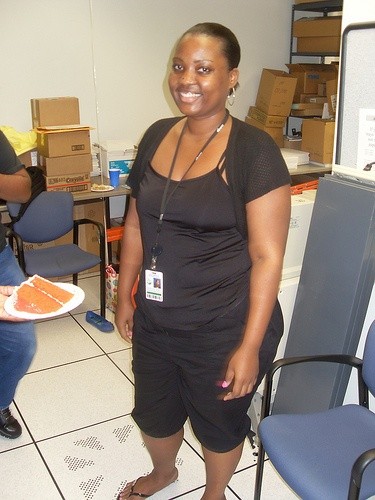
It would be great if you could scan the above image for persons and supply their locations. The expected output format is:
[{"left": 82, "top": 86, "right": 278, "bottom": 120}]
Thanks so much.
[
  {"left": 0, "top": 129, "right": 38, "bottom": 439},
  {"left": 154, "top": 280, "right": 161, "bottom": 288},
  {"left": 116, "top": 24, "right": 293, "bottom": 500}
]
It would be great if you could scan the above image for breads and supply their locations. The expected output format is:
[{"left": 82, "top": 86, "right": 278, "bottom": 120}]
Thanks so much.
[{"left": 92, "top": 184, "right": 109, "bottom": 190}]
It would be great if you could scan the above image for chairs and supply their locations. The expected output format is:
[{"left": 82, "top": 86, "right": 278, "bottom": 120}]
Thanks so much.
[
  {"left": 6, "top": 190, "right": 106, "bottom": 318},
  {"left": 253, "top": 321, "right": 375, "bottom": 500}
]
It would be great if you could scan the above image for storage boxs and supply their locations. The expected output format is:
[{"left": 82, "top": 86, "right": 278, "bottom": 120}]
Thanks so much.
[
  {"left": 30, "top": 96, "right": 92, "bottom": 195},
  {"left": 244, "top": 16, "right": 341, "bottom": 163}
]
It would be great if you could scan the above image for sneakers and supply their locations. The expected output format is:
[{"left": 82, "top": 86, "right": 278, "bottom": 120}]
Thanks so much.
[{"left": 0, "top": 409, "right": 21, "bottom": 439}]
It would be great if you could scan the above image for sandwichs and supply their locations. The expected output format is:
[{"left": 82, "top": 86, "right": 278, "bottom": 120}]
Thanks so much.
[{"left": 12, "top": 274, "right": 74, "bottom": 313}]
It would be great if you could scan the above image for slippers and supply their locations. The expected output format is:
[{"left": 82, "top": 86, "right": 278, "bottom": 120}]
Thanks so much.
[{"left": 116, "top": 467, "right": 178, "bottom": 500}]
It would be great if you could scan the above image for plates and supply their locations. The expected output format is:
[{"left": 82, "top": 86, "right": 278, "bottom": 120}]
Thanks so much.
[
  {"left": 3, "top": 282, "right": 85, "bottom": 319},
  {"left": 91, "top": 185, "right": 114, "bottom": 192}
]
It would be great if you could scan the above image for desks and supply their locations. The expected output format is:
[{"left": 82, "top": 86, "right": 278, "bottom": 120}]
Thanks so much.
[{"left": 0, "top": 189, "right": 130, "bottom": 266}]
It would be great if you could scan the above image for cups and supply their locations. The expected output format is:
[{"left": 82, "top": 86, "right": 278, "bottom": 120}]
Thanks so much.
[{"left": 109, "top": 169, "right": 120, "bottom": 189}]
